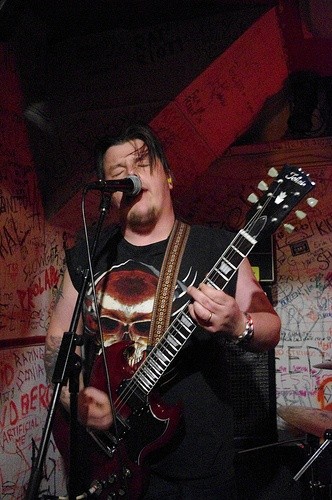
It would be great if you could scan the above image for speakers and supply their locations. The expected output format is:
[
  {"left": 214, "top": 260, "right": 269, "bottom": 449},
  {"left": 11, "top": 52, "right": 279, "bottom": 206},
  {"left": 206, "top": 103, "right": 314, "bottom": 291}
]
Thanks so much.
[{"left": 212, "top": 227, "right": 279, "bottom": 454}]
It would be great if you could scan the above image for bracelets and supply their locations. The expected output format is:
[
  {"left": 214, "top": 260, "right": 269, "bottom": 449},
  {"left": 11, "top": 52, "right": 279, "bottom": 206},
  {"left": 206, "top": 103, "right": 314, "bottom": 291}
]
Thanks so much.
[{"left": 226, "top": 311, "right": 254, "bottom": 347}]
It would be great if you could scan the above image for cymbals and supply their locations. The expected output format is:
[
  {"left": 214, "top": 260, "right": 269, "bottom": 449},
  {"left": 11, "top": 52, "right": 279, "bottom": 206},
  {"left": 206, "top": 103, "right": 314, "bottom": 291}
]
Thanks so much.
[
  {"left": 276, "top": 406, "right": 332, "bottom": 439},
  {"left": 314, "top": 360, "right": 332, "bottom": 371}
]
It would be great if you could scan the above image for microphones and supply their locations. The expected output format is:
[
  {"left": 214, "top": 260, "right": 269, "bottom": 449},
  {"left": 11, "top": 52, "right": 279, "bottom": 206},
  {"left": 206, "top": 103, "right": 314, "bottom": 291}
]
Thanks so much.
[{"left": 86, "top": 176, "right": 142, "bottom": 199}]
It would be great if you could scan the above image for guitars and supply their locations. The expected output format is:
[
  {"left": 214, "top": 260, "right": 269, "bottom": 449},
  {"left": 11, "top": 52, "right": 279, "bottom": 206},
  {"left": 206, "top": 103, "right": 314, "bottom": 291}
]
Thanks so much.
[{"left": 80, "top": 162, "right": 321, "bottom": 481}]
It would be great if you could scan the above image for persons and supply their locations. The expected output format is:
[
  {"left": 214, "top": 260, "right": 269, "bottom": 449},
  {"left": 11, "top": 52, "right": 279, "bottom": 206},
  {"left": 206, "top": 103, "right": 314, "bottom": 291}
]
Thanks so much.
[{"left": 43, "top": 122, "right": 281, "bottom": 500}]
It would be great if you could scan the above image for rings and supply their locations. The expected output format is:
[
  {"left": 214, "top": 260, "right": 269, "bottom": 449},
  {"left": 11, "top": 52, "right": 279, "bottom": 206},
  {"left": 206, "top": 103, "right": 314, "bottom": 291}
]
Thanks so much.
[{"left": 204, "top": 313, "right": 213, "bottom": 323}]
